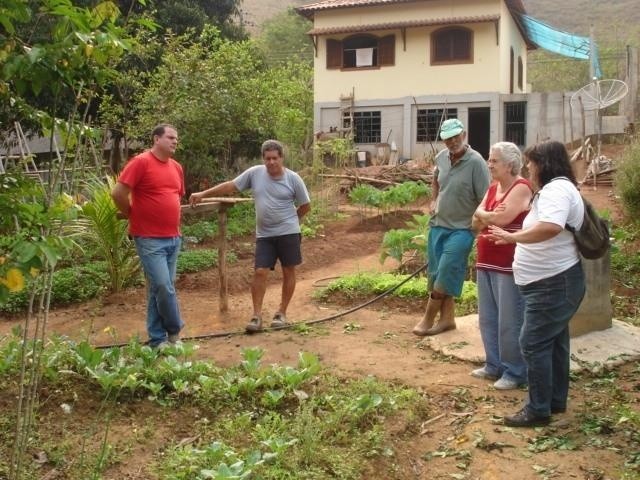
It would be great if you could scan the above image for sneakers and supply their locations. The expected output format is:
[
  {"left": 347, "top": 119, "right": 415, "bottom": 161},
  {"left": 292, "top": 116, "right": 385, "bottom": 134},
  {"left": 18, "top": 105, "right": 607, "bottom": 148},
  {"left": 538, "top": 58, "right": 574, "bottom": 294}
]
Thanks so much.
[
  {"left": 271, "top": 312, "right": 285, "bottom": 326},
  {"left": 158, "top": 340, "right": 182, "bottom": 351},
  {"left": 494, "top": 378, "right": 518, "bottom": 389},
  {"left": 247, "top": 316, "right": 261, "bottom": 330},
  {"left": 472, "top": 364, "right": 495, "bottom": 379}
]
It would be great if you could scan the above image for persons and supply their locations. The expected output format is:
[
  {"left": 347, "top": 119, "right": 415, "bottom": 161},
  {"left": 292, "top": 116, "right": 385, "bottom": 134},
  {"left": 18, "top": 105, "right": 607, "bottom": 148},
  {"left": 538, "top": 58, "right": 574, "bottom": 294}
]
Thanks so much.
[
  {"left": 111, "top": 123, "right": 186, "bottom": 356},
  {"left": 471, "top": 141, "right": 534, "bottom": 391},
  {"left": 412, "top": 118, "right": 491, "bottom": 337},
  {"left": 483, "top": 139, "right": 586, "bottom": 427},
  {"left": 189, "top": 139, "right": 311, "bottom": 332}
]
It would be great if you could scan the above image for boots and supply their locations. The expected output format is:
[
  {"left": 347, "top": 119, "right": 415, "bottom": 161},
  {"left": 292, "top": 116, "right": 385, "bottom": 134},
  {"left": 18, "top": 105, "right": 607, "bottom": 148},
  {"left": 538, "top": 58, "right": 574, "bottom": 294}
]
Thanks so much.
[
  {"left": 427, "top": 297, "right": 456, "bottom": 335},
  {"left": 413, "top": 297, "right": 441, "bottom": 336}
]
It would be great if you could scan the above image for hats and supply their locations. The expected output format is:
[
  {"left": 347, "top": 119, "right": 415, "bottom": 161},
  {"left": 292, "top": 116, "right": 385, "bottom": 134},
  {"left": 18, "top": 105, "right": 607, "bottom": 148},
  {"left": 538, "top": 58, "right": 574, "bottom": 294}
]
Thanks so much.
[{"left": 440, "top": 120, "right": 463, "bottom": 140}]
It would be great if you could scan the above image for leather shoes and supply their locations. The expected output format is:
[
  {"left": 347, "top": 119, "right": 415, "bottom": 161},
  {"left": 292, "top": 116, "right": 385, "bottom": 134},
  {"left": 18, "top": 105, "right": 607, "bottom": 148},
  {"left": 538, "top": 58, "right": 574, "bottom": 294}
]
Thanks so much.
[{"left": 505, "top": 409, "right": 549, "bottom": 426}]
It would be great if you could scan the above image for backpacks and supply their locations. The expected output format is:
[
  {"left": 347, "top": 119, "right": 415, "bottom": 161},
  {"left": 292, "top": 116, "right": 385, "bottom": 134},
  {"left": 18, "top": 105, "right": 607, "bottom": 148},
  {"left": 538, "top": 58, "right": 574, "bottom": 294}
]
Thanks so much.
[{"left": 538, "top": 178, "right": 608, "bottom": 259}]
